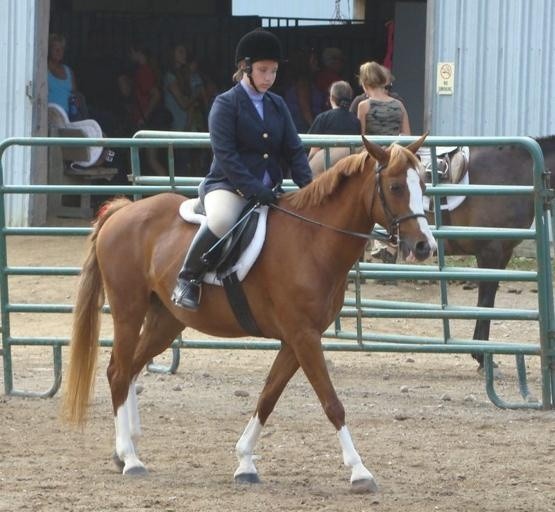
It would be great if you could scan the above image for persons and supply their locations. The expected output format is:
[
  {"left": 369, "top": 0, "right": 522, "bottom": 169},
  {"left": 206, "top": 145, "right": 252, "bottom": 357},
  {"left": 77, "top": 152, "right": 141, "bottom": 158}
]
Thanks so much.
[
  {"left": 317, "top": 48, "right": 353, "bottom": 95},
  {"left": 302, "top": 81, "right": 360, "bottom": 180},
  {"left": 357, "top": 62, "right": 412, "bottom": 151},
  {"left": 157, "top": 37, "right": 198, "bottom": 177},
  {"left": 171, "top": 30, "right": 312, "bottom": 311},
  {"left": 47, "top": 22, "right": 165, "bottom": 203},
  {"left": 282, "top": 47, "right": 321, "bottom": 133}
]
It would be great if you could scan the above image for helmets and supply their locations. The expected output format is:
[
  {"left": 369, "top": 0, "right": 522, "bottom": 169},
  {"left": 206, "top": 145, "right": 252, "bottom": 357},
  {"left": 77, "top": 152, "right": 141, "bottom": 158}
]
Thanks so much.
[{"left": 236, "top": 30, "right": 284, "bottom": 63}]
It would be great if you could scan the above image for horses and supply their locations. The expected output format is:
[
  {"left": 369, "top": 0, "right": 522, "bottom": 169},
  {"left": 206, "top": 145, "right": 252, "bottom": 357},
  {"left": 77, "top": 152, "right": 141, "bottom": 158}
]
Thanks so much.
[
  {"left": 309, "top": 133, "right": 555, "bottom": 378},
  {"left": 63, "top": 129, "right": 440, "bottom": 492}
]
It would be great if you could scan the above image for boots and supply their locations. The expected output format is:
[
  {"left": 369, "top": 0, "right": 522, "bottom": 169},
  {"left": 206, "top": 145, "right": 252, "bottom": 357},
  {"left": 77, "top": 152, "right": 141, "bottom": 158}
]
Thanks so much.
[{"left": 171, "top": 221, "right": 227, "bottom": 309}]
